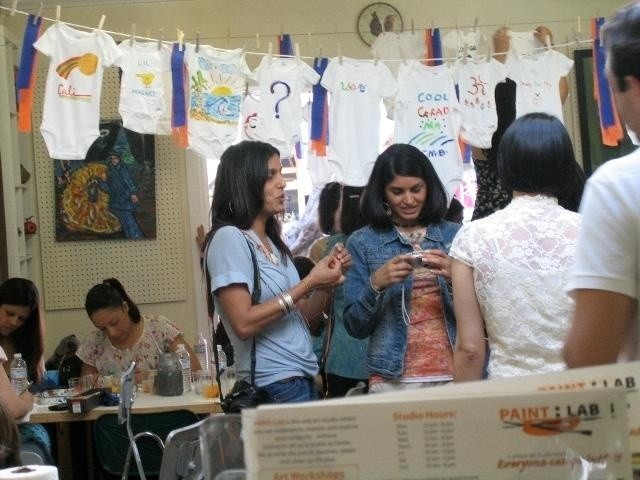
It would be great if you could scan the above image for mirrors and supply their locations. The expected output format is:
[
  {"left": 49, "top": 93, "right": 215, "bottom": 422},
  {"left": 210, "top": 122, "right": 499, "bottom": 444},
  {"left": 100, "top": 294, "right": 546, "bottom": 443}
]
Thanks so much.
[{"left": 574, "top": 46, "right": 640, "bottom": 182}]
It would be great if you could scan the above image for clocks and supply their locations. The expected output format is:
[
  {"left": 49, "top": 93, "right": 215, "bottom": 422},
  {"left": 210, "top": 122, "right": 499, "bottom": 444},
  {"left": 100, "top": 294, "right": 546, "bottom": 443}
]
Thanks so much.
[{"left": 356, "top": 2, "right": 405, "bottom": 48}]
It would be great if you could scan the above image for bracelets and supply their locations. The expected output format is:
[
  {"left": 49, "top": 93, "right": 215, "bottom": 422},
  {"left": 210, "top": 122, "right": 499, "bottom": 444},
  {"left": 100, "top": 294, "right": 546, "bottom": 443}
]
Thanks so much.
[
  {"left": 274, "top": 295, "right": 288, "bottom": 315},
  {"left": 280, "top": 291, "right": 294, "bottom": 312},
  {"left": 301, "top": 279, "right": 309, "bottom": 296}
]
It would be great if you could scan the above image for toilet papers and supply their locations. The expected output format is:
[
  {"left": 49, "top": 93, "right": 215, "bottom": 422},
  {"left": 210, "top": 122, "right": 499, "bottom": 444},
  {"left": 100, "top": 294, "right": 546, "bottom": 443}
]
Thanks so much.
[{"left": 0, "top": 464, "right": 59, "bottom": 480}]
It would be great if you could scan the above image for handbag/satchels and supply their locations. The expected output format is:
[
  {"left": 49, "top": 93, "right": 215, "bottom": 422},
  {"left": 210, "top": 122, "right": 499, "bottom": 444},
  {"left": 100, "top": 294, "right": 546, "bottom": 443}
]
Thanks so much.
[{"left": 205, "top": 225, "right": 267, "bottom": 414}]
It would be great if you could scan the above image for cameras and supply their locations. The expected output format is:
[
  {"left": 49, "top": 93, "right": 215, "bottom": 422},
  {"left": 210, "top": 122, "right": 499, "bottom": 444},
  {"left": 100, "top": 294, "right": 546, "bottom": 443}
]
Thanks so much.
[{"left": 405, "top": 251, "right": 430, "bottom": 270}]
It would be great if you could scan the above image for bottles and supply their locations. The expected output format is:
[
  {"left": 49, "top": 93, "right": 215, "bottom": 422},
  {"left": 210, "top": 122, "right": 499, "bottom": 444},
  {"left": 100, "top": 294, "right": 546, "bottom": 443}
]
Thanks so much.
[
  {"left": 176, "top": 344, "right": 192, "bottom": 393},
  {"left": 193, "top": 333, "right": 209, "bottom": 372},
  {"left": 158, "top": 352, "right": 183, "bottom": 396},
  {"left": 10, "top": 353, "right": 27, "bottom": 397},
  {"left": 210, "top": 344, "right": 228, "bottom": 388}
]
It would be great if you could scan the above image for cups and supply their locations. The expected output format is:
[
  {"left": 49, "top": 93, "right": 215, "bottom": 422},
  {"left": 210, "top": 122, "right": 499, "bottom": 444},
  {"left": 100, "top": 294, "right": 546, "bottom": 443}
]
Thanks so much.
[
  {"left": 83, "top": 375, "right": 123, "bottom": 393},
  {"left": 192, "top": 372, "right": 211, "bottom": 395},
  {"left": 143, "top": 370, "right": 159, "bottom": 393},
  {"left": 68, "top": 378, "right": 81, "bottom": 396},
  {"left": 200, "top": 375, "right": 219, "bottom": 398}
]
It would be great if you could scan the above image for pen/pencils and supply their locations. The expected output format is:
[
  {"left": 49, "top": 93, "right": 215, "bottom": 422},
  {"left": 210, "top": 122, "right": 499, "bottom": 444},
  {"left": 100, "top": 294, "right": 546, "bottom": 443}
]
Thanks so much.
[
  {"left": 19, "top": 381, "right": 34, "bottom": 395},
  {"left": 91, "top": 367, "right": 106, "bottom": 389}
]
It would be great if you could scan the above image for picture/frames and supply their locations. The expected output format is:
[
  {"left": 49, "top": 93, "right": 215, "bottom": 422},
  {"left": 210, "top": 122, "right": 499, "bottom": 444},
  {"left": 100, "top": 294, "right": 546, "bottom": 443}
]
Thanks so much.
[{"left": 52, "top": 120, "right": 158, "bottom": 243}]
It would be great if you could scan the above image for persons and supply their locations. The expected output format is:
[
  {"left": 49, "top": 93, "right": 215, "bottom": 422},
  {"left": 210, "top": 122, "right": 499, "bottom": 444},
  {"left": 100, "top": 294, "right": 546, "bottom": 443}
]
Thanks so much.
[
  {"left": 452, "top": 112, "right": 584, "bottom": 384},
  {"left": 207, "top": 141, "right": 352, "bottom": 404},
  {"left": 195, "top": 225, "right": 234, "bottom": 369},
  {"left": 561, "top": 2, "right": 640, "bottom": 369},
  {"left": 293, "top": 257, "right": 327, "bottom": 399},
  {"left": 0, "top": 343, "right": 50, "bottom": 470},
  {"left": 0, "top": 278, "right": 52, "bottom": 466},
  {"left": 73, "top": 277, "right": 203, "bottom": 480},
  {"left": 342, "top": 143, "right": 465, "bottom": 394},
  {"left": 323, "top": 186, "right": 373, "bottom": 397},
  {"left": 309, "top": 180, "right": 344, "bottom": 263},
  {"left": 85, "top": 152, "right": 143, "bottom": 239}
]
